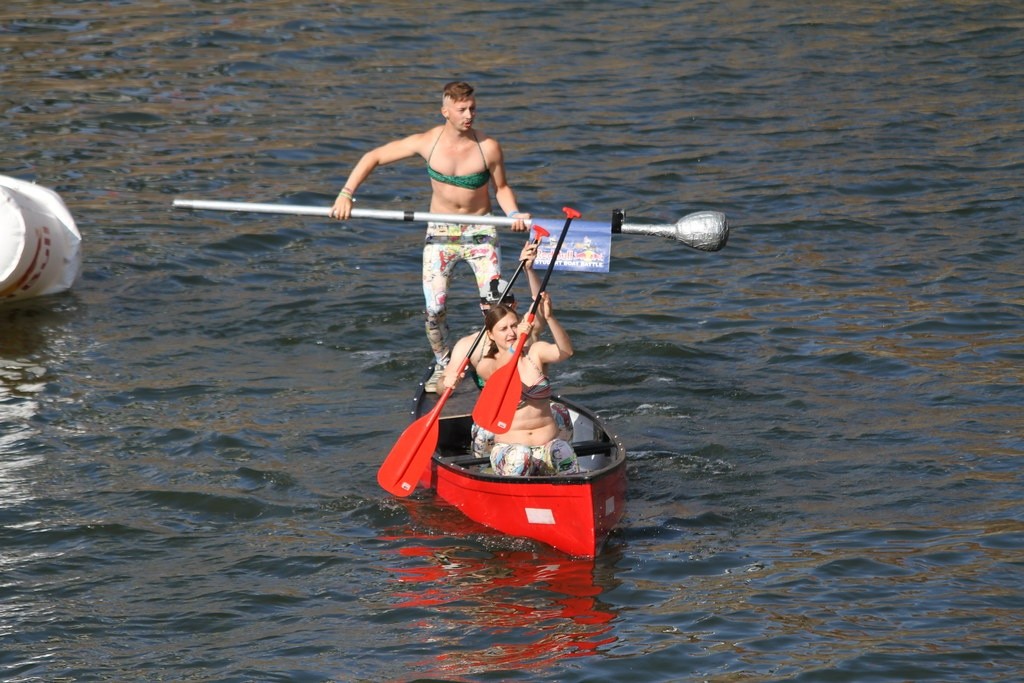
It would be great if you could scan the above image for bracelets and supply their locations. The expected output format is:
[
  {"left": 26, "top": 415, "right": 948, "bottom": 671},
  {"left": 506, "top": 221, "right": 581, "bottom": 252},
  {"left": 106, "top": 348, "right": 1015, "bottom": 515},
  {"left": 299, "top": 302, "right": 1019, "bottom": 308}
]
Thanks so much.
[
  {"left": 338, "top": 186, "right": 356, "bottom": 203},
  {"left": 509, "top": 211, "right": 520, "bottom": 217},
  {"left": 509, "top": 345, "right": 515, "bottom": 354}
]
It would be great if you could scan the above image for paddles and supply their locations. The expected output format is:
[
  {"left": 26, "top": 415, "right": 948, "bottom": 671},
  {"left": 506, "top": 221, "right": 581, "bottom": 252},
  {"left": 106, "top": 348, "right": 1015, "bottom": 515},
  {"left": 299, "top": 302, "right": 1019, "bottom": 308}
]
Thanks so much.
[
  {"left": 375, "top": 225, "right": 550, "bottom": 498},
  {"left": 471, "top": 207, "right": 581, "bottom": 436}
]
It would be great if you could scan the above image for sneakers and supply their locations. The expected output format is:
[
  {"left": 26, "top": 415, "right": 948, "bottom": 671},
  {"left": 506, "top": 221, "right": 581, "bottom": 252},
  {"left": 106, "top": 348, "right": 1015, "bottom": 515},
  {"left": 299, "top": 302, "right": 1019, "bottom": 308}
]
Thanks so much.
[{"left": 424, "top": 361, "right": 450, "bottom": 392}]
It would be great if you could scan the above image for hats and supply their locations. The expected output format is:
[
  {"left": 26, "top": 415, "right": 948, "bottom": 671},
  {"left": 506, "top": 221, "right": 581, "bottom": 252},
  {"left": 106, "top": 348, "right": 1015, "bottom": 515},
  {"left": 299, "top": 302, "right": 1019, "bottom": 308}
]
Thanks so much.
[{"left": 480, "top": 278, "right": 515, "bottom": 304}]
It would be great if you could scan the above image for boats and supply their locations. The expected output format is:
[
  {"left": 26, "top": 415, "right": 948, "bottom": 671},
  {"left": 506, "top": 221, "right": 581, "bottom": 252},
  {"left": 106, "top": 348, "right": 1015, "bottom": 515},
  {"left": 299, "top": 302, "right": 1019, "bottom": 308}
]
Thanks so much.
[{"left": 412, "top": 355, "right": 622, "bottom": 559}]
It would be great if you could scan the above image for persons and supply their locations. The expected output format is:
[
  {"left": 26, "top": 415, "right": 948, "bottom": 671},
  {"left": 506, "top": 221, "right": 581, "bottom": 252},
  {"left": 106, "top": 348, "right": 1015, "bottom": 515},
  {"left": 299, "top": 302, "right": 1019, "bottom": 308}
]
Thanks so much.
[
  {"left": 437, "top": 238, "right": 578, "bottom": 475},
  {"left": 329, "top": 82, "right": 531, "bottom": 393}
]
